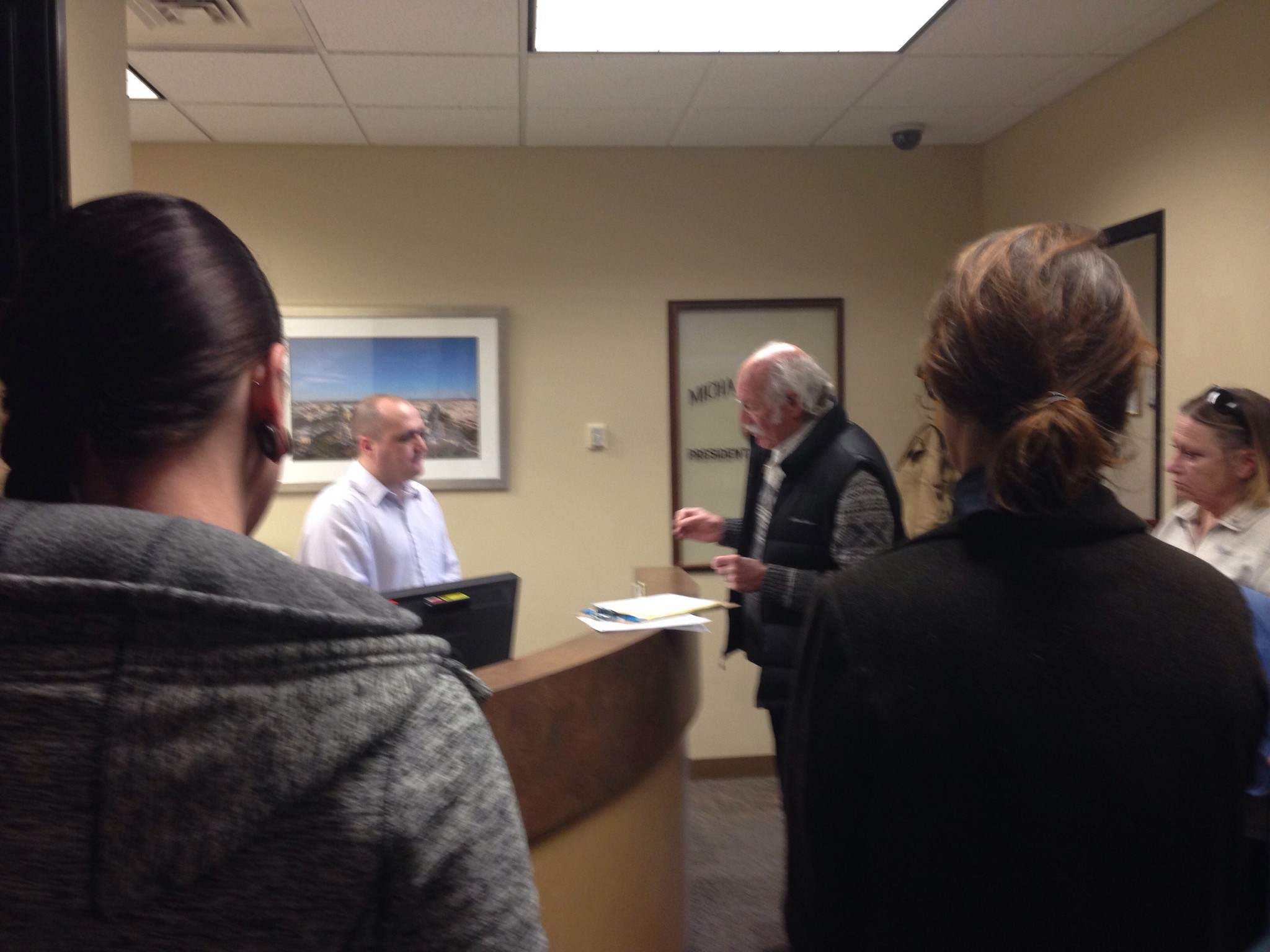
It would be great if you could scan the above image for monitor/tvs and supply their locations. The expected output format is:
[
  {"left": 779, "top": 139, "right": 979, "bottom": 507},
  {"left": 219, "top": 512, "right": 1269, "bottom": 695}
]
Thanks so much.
[{"left": 378, "top": 572, "right": 520, "bottom": 669}]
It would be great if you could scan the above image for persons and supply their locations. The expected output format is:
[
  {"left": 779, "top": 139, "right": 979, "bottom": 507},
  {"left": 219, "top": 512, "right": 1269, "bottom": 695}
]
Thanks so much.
[
  {"left": 0, "top": 192, "right": 546, "bottom": 952},
  {"left": 681, "top": 338, "right": 910, "bottom": 664},
  {"left": 758, "top": 223, "right": 1270, "bottom": 952},
  {"left": 298, "top": 394, "right": 463, "bottom": 609},
  {"left": 1149, "top": 386, "right": 1270, "bottom": 682}
]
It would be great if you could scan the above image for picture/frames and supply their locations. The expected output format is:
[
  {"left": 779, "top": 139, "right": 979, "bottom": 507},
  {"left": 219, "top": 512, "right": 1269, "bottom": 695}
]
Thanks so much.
[
  {"left": 273, "top": 306, "right": 508, "bottom": 493},
  {"left": 668, "top": 297, "right": 845, "bottom": 573},
  {"left": 1096, "top": 208, "right": 1165, "bottom": 523}
]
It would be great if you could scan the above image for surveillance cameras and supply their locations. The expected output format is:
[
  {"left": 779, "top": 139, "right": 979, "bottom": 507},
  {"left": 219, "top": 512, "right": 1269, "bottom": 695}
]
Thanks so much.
[{"left": 890, "top": 122, "right": 924, "bottom": 151}]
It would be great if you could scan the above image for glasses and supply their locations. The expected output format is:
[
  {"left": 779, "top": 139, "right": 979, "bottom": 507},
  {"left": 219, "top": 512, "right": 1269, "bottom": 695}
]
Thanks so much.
[
  {"left": 1207, "top": 385, "right": 1249, "bottom": 430},
  {"left": 735, "top": 398, "right": 790, "bottom": 417}
]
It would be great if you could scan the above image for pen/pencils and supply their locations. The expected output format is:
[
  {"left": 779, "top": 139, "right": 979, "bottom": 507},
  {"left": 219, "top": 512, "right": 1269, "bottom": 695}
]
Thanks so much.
[{"left": 580, "top": 608, "right": 644, "bottom": 623}]
